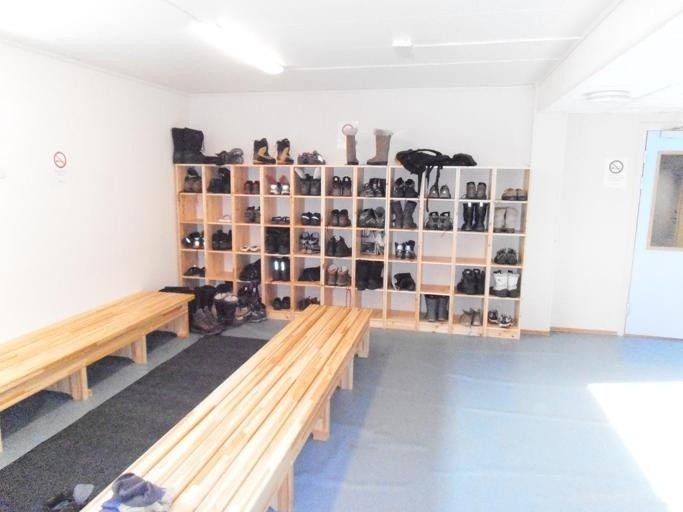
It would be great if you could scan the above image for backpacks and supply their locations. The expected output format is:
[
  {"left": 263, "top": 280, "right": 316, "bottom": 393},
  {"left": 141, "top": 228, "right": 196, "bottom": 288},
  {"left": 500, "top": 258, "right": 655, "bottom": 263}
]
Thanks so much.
[{"left": 395, "top": 148, "right": 476, "bottom": 212}]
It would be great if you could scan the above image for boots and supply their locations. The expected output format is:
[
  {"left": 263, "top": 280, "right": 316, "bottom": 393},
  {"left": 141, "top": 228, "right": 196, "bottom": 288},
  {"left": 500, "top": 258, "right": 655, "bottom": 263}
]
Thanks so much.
[
  {"left": 170, "top": 123, "right": 392, "bottom": 166},
  {"left": 181, "top": 168, "right": 525, "bottom": 335}
]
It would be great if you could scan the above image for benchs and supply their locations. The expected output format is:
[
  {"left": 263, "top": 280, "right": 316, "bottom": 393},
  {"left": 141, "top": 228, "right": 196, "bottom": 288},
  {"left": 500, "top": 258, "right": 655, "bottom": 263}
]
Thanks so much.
[
  {"left": 0, "top": 290, "right": 195, "bottom": 453},
  {"left": 80, "top": 304, "right": 373, "bottom": 512}
]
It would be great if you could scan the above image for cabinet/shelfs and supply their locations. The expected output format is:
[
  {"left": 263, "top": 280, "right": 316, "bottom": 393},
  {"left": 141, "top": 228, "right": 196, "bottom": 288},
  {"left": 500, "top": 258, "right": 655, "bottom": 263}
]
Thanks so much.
[{"left": 173, "top": 163, "right": 532, "bottom": 340}]
